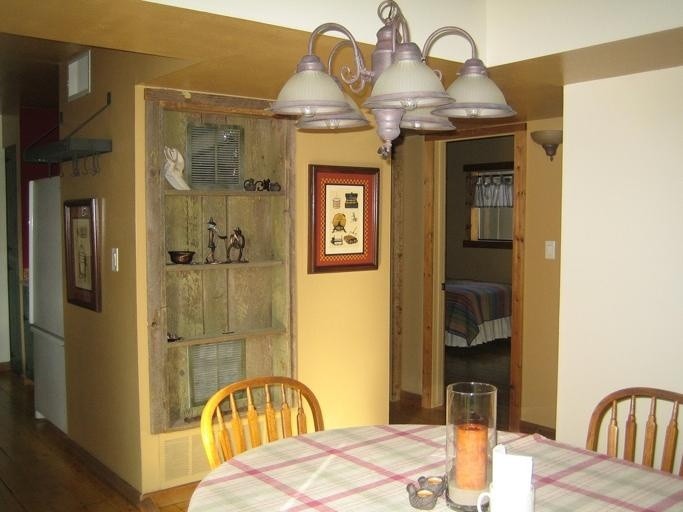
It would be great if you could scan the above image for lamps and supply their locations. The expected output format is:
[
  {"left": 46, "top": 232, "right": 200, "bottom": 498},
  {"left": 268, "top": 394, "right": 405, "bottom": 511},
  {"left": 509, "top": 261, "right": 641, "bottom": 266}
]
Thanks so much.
[
  {"left": 529, "top": 129, "right": 561, "bottom": 160},
  {"left": 271, "top": 0, "right": 516, "bottom": 159}
]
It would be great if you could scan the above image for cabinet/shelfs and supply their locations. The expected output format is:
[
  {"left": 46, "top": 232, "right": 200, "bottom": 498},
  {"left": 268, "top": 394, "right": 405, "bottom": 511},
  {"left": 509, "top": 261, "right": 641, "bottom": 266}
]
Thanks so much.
[{"left": 142, "top": 86, "right": 297, "bottom": 435}]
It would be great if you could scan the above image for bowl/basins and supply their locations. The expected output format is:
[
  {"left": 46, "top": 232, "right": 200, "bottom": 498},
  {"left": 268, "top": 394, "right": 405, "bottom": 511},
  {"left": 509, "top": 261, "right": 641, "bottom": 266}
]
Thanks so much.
[{"left": 168, "top": 251, "right": 197, "bottom": 265}]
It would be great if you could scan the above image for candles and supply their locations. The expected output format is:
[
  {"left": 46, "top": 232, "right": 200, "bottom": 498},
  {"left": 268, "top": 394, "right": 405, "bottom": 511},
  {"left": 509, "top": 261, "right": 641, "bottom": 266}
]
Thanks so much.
[{"left": 455, "top": 423, "right": 487, "bottom": 491}]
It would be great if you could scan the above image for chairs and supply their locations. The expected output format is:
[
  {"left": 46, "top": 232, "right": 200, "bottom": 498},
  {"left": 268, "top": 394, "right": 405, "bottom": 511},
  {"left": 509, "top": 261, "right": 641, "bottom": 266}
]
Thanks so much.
[
  {"left": 584, "top": 386, "right": 682, "bottom": 478},
  {"left": 199, "top": 375, "right": 323, "bottom": 468}
]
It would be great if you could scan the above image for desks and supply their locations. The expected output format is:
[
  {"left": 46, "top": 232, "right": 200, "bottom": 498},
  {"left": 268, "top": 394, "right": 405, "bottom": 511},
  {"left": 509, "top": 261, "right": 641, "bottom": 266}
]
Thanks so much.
[{"left": 187, "top": 423, "right": 683, "bottom": 512}]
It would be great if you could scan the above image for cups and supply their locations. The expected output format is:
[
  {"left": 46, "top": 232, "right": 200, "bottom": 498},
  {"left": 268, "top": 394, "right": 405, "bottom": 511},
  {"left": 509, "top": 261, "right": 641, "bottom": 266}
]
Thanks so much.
[
  {"left": 477, "top": 484, "right": 535, "bottom": 511},
  {"left": 447, "top": 383, "right": 497, "bottom": 512}
]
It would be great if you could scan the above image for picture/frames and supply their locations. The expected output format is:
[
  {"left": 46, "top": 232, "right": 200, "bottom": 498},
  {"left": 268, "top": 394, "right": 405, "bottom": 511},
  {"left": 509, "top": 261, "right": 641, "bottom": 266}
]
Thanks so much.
[
  {"left": 61, "top": 196, "right": 102, "bottom": 314},
  {"left": 306, "top": 163, "right": 380, "bottom": 275}
]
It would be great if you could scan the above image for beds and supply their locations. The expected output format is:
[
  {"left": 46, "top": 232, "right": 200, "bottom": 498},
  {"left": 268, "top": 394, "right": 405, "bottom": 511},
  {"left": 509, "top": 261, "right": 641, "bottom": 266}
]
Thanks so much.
[{"left": 444, "top": 280, "right": 512, "bottom": 365}]
